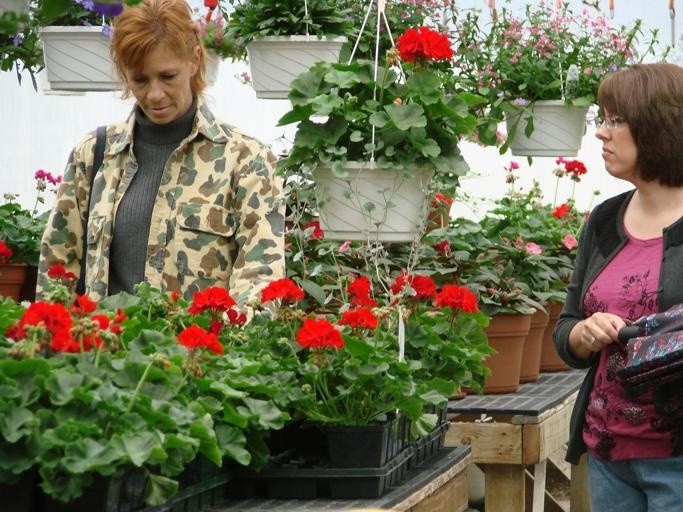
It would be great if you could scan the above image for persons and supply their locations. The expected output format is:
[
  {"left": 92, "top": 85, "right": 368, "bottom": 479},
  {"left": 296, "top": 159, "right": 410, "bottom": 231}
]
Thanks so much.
[
  {"left": 33, "top": 2, "right": 289, "bottom": 321},
  {"left": 550, "top": 60, "right": 682, "bottom": 511}
]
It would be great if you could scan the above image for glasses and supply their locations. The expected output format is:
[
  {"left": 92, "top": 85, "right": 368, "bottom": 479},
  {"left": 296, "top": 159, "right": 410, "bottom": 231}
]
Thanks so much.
[{"left": 594, "top": 114, "right": 627, "bottom": 130}]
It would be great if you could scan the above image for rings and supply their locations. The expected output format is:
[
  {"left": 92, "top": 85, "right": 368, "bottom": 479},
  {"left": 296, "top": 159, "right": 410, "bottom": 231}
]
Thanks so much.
[{"left": 589, "top": 336, "right": 597, "bottom": 346}]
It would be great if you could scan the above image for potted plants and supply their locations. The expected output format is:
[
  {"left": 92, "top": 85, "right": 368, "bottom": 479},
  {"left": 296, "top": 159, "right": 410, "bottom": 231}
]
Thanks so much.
[
  {"left": 3, "top": 275, "right": 500, "bottom": 482},
  {"left": 0, "top": 158, "right": 604, "bottom": 394},
  {"left": 225, "top": 1, "right": 349, "bottom": 101}
]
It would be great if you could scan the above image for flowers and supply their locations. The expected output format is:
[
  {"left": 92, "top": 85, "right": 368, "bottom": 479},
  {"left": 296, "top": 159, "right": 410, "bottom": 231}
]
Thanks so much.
[
  {"left": 461, "top": 1, "right": 666, "bottom": 155},
  {"left": 273, "top": 26, "right": 497, "bottom": 182},
  {"left": 2, "top": 0, "right": 146, "bottom": 91}
]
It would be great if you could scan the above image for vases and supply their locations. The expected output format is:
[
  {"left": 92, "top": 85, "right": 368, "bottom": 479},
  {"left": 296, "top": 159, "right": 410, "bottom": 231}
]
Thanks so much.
[
  {"left": 301, "top": 159, "right": 440, "bottom": 244},
  {"left": 7, "top": 404, "right": 452, "bottom": 508},
  {"left": 494, "top": 93, "right": 593, "bottom": 158},
  {"left": 37, "top": 23, "right": 136, "bottom": 92}
]
